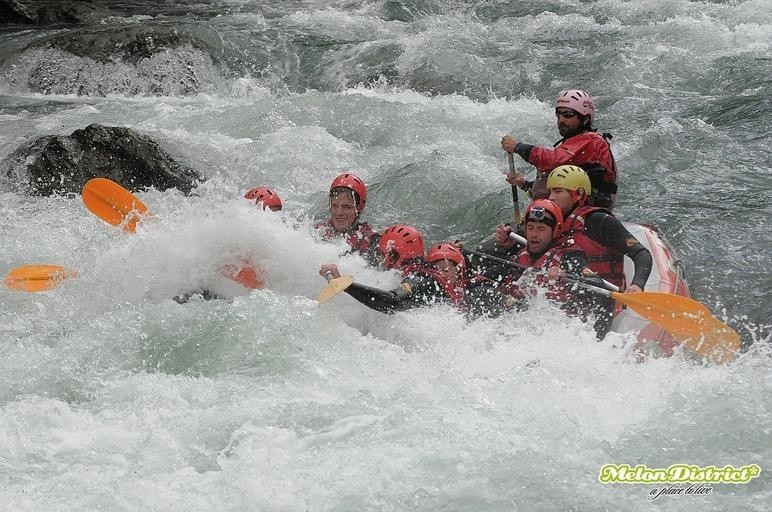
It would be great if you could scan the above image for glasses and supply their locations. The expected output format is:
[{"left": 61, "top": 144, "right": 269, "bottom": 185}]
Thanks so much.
[{"left": 555, "top": 109, "right": 577, "bottom": 118}]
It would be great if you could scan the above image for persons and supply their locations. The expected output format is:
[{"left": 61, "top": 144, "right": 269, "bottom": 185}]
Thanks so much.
[
  {"left": 497, "top": 198, "right": 609, "bottom": 342},
  {"left": 495, "top": 165, "right": 653, "bottom": 310},
  {"left": 313, "top": 174, "right": 373, "bottom": 256},
  {"left": 501, "top": 89, "right": 618, "bottom": 212},
  {"left": 423, "top": 243, "right": 507, "bottom": 321},
  {"left": 319, "top": 225, "right": 465, "bottom": 315}
]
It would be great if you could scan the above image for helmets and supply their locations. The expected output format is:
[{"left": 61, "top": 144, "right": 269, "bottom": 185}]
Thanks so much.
[
  {"left": 525, "top": 198, "right": 563, "bottom": 239},
  {"left": 546, "top": 164, "right": 592, "bottom": 196},
  {"left": 330, "top": 173, "right": 367, "bottom": 213},
  {"left": 377, "top": 224, "right": 425, "bottom": 264},
  {"left": 554, "top": 89, "right": 595, "bottom": 125},
  {"left": 427, "top": 243, "right": 466, "bottom": 272}
]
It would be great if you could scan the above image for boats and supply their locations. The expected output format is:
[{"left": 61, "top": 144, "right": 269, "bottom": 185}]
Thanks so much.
[{"left": 602, "top": 217, "right": 690, "bottom": 363}]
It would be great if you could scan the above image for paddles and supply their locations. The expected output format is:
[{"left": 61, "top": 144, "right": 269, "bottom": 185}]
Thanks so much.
[
  {"left": 464, "top": 245, "right": 713, "bottom": 336},
  {"left": 319, "top": 266, "right": 354, "bottom": 304},
  {"left": 83, "top": 179, "right": 151, "bottom": 235},
  {"left": 509, "top": 231, "right": 742, "bottom": 361},
  {"left": 5, "top": 265, "right": 64, "bottom": 292}
]
